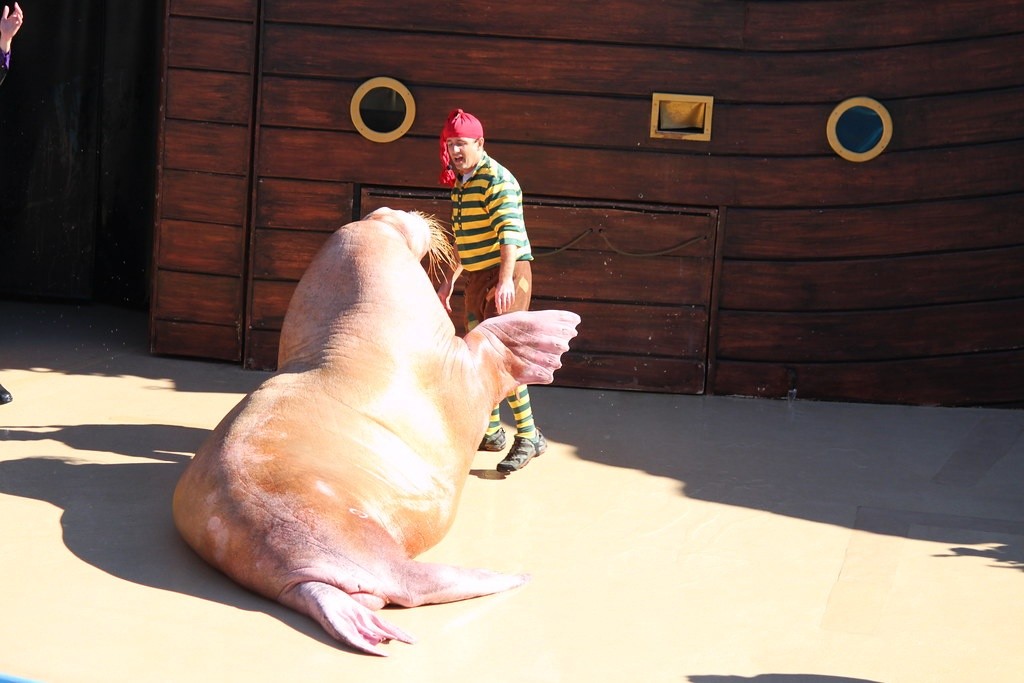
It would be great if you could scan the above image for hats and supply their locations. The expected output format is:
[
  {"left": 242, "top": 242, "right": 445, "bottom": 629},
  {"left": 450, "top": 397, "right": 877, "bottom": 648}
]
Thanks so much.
[{"left": 440, "top": 109, "right": 483, "bottom": 188}]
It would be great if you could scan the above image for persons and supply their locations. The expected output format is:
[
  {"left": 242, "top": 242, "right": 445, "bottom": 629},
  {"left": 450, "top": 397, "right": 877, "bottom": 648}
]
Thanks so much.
[
  {"left": 0, "top": 2, "right": 23, "bottom": 403},
  {"left": 438, "top": 108, "right": 546, "bottom": 472}
]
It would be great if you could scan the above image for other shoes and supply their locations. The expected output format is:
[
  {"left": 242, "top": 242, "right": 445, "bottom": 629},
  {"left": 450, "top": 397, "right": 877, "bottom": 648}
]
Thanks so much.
[
  {"left": 497, "top": 428, "right": 547, "bottom": 472},
  {"left": 477, "top": 427, "right": 506, "bottom": 451}
]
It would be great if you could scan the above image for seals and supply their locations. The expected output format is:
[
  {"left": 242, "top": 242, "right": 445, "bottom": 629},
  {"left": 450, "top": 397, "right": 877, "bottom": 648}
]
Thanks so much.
[{"left": 168, "top": 205, "right": 585, "bottom": 657}]
